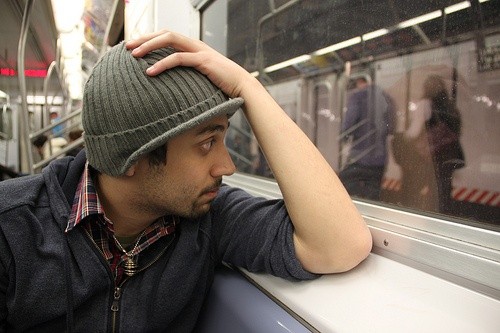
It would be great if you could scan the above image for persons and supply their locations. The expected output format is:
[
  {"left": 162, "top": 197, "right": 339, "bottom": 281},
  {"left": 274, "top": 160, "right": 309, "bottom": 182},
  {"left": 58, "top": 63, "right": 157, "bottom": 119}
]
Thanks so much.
[
  {"left": 0, "top": 28, "right": 373, "bottom": 333},
  {"left": 32, "top": 129, "right": 84, "bottom": 173},
  {"left": 337, "top": 69, "right": 398, "bottom": 202},
  {"left": 403, "top": 74, "right": 466, "bottom": 215}
]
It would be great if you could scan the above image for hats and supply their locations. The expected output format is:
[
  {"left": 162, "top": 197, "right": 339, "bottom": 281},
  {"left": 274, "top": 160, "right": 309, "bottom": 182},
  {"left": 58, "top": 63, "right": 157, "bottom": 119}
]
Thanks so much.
[
  {"left": 346, "top": 66, "right": 374, "bottom": 90},
  {"left": 418, "top": 75, "right": 445, "bottom": 99},
  {"left": 83, "top": 40, "right": 244, "bottom": 176}
]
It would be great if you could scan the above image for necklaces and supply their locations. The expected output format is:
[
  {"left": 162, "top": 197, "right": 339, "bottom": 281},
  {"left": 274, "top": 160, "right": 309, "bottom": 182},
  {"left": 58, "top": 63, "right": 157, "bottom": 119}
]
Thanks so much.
[{"left": 97, "top": 218, "right": 150, "bottom": 277}]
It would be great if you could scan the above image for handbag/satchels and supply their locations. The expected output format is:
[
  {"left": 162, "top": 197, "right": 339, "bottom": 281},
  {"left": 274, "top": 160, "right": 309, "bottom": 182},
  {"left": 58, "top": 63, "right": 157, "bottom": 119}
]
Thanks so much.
[{"left": 431, "top": 141, "right": 465, "bottom": 174}]
